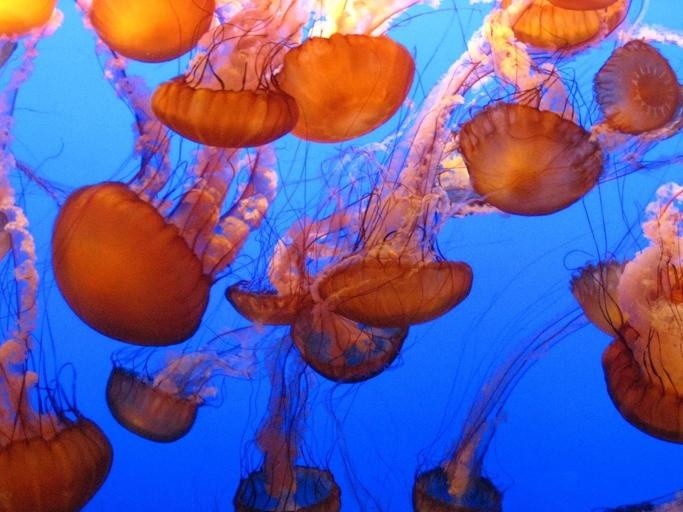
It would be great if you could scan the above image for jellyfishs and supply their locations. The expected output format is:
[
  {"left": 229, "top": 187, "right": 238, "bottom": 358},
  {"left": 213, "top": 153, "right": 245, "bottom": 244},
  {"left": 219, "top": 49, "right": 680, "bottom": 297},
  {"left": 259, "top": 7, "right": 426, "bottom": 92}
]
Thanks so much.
[{"left": 0, "top": 0, "right": 683, "bottom": 512}]
